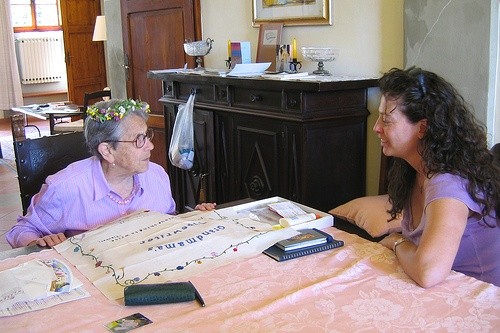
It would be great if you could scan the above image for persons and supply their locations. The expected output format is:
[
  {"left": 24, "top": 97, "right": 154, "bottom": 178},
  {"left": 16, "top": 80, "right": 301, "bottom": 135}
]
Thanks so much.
[
  {"left": 6, "top": 99, "right": 216, "bottom": 247},
  {"left": 373, "top": 66, "right": 500, "bottom": 289}
]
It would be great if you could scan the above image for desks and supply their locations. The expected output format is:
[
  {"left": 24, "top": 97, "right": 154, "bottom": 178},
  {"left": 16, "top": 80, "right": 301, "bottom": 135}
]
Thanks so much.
[
  {"left": 0, "top": 197, "right": 500, "bottom": 333},
  {"left": 9, "top": 102, "right": 83, "bottom": 141}
]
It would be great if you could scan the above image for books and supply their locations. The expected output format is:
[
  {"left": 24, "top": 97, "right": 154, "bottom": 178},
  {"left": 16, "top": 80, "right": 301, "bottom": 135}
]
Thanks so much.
[
  {"left": 201, "top": 62, "right": 308, "bottom": 79},
  {"left": 262, "top": 229, "right": 344, "bottom": 262},
  {"left": 256, "top": 24, "right": 283, "bottom": 72},
  {"left": 230, "top": 42, "right": 251, "bottom": 69}
]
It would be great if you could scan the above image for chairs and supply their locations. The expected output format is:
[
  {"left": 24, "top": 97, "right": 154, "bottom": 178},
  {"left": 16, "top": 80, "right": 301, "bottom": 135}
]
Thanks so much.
[
  {"left": 47, "top": 91, "right": 112, "bottom": 135},
  {"left": 13, "top": 132, "right": 92, "bottom": 216}
]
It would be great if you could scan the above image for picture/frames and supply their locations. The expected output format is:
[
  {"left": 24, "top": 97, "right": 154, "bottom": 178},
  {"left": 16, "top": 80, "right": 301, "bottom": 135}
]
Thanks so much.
[{"left": 252, "top": 0, "right": 334, "bottom": 28}]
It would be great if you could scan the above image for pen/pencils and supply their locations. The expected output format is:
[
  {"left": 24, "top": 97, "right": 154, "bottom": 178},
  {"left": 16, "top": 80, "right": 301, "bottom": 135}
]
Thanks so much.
[{"left": 188, "top": 280, "right": 205, "bottom": 307}]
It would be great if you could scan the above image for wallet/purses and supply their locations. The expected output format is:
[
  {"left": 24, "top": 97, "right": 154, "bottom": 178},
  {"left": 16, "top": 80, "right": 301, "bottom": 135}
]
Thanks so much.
[{"left": 124, "top": 282, "right": 194, "bottom": 306}]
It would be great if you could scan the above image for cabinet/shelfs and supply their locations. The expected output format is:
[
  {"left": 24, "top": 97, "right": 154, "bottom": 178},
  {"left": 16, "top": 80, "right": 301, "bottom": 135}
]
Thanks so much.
[{"left": 147, "top": 69, "right": 381, "bottom": 217}]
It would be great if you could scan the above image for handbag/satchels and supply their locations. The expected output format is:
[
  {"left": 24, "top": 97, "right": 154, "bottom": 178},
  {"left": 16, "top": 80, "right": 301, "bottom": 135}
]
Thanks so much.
[{"left": 168, "top": 92, "right": 195, "bottom": 170}]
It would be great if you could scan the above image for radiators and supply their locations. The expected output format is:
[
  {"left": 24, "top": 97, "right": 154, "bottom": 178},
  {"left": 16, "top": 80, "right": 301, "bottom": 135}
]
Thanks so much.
[{"left": 15, "top": 34, "right": 64, "bottom": 84}]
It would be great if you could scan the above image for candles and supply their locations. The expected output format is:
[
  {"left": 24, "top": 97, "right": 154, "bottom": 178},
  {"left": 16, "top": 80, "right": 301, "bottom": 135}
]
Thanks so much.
[{"left": 293, "top": 38, "right": 297, "bottom": 58}]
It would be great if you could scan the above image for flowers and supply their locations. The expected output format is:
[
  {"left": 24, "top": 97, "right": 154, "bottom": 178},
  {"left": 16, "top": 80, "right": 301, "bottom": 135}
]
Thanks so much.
[{"left": 86, "top": 98, "right": 151, "bottom": 123}]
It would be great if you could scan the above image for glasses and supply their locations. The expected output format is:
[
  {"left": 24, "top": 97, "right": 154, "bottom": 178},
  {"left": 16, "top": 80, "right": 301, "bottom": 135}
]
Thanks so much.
[{"left": 100, "top": 128, "right": 154, "bottom": 148}]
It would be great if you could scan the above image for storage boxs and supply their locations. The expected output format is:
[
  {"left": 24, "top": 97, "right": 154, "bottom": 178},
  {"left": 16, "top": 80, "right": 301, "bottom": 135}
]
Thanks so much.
[{"left": 218, "top": 196, "right": 334, "bottom": 230}]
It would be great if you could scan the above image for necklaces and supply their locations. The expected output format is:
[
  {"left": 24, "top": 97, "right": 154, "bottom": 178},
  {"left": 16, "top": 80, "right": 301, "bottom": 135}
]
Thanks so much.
[{"left": 107, "top": 194, "right": 135, "bottom": 204}]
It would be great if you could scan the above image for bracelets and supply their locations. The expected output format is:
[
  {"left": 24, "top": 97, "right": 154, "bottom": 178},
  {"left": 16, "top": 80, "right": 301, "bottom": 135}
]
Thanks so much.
[{"left": 393, "top": 238, "right": 412, "bottom": 255}]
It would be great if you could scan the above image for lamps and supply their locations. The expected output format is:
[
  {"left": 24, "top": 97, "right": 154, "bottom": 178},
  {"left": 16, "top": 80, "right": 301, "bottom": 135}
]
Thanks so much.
[{"left": 92, "top": 15, "right": 107, "bottom": 41}]
